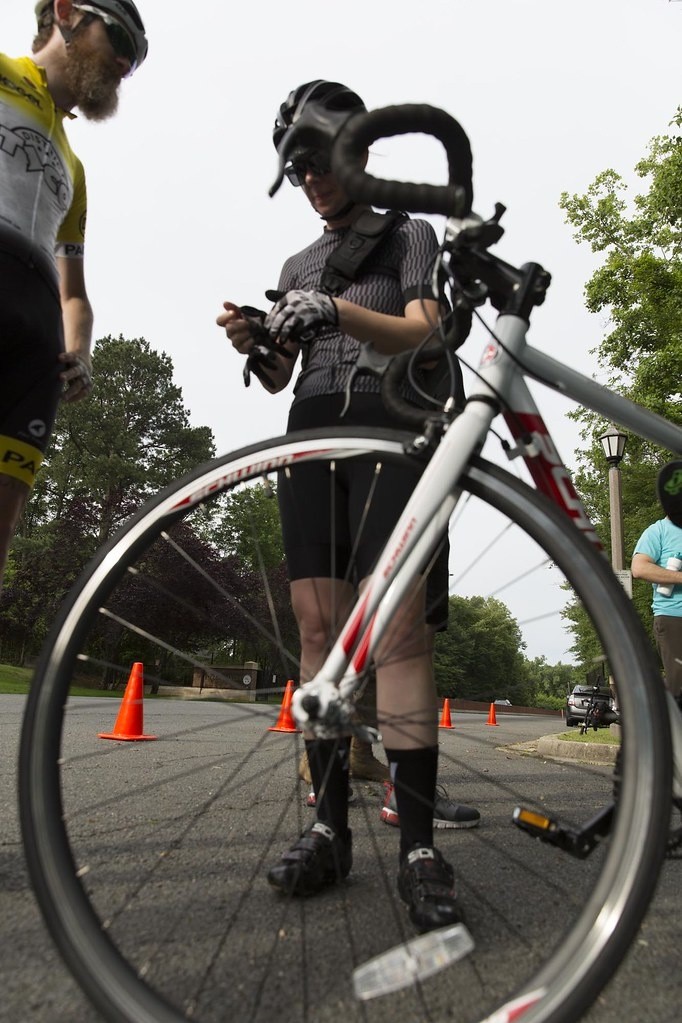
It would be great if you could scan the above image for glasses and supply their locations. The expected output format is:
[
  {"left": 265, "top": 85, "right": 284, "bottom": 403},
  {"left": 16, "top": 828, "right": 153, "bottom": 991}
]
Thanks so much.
[
  {"left": 285, "top": 143, "right": 337, "bottom": 187},
  {"left": 71, "top": 3, "right": 138, "bottom": 78}
]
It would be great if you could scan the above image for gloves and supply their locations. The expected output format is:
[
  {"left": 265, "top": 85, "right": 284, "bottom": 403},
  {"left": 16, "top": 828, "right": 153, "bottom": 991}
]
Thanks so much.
[
  {"left": 236, "top": 305, "right": 295, "bottom": 395},
  {"left": 263, "top": 289, "right": 340, "bottom": 345}
]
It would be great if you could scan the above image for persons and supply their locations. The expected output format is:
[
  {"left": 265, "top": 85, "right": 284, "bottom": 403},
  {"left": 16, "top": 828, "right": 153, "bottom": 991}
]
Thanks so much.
[
  {"left": 214, "top": 80, "right": 480, "bottom": 933},
  {"left": 630, "top": 515, "right": 682, "bottom": 713},
  {"left": 0, "top": 0, "right": 148, "bottom": 564}
]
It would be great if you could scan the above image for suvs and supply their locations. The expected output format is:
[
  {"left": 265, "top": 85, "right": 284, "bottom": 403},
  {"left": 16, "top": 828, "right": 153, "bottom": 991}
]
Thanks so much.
[{"left": 566, "top": 685, "right": 615, "bottom": 728}]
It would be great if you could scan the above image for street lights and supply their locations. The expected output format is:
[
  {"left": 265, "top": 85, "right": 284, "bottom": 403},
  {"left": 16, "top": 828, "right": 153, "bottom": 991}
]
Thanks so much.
[{"left": 596, "top": 421, "right": 627, "bottom": 574}]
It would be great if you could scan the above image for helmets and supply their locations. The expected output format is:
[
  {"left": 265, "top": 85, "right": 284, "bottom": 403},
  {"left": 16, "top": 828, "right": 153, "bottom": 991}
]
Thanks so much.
[
  {"left": 272, "top": 78, "right": 369, "bottom": 158},
  {"left": 35, "top": 0, "right": 148, "bottom": 68}
]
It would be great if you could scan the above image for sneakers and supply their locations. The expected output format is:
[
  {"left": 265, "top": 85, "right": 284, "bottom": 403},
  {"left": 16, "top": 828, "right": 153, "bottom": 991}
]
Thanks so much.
[
  {"left": 380, "top": 780, "right": 480, "bottom": 828},
  {"left": 266, "top": 823, "right": 353, "bottom": 890},
  {"left": 306, "top": 786, "right": 358, "bottom": 804},
  {"left": 396, "top": 843, "right": 466, "bottom": 932}
]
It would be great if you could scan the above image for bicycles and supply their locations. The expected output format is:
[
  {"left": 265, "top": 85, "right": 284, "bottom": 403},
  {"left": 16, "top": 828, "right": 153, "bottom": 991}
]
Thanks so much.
[{"left": 13, "top": 98, "right": 682, "bottom": 1023}]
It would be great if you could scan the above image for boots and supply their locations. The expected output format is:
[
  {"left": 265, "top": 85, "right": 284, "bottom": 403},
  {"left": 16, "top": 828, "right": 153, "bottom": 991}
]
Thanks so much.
[{"left": 352, "top": 742, "right": 394, "bottom": 783}]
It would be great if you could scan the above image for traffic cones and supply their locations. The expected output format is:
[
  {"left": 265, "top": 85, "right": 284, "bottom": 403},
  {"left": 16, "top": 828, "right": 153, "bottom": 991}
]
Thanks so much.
[
  {"left": 439, "top": 699, "right": 455, "bottom": 729},
  {"left": 485, "top": 703, "right": 502, "bottom": 730},
  {"left": 98, "top": 662, "right": 157, "bottom": 744},
  {"left": 267, "top": 679, "right": 303, "bottom": 733}
]
